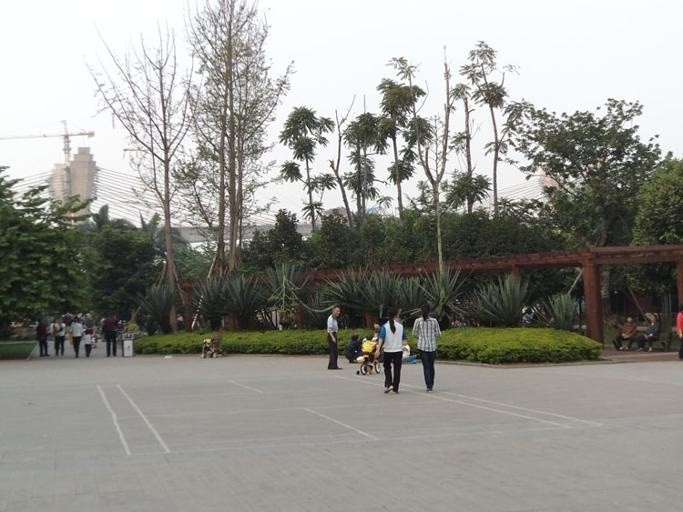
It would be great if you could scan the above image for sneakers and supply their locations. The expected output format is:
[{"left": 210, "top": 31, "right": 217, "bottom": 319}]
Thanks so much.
[{"left": 384, "top": 385, "right": 393, "bottom": 393}]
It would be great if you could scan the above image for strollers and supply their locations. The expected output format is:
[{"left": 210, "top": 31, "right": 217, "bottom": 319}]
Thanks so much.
[
  {"left": 356, "top": 337, "right": 383, "bottom": 375},
  {"left": 200, "top": 328, "right": 227, "bottom": 360}
]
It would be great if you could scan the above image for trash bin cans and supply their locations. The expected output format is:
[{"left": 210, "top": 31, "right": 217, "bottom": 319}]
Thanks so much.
[{"left": 121, "top": 332, "right": 134, "bottom": 357}]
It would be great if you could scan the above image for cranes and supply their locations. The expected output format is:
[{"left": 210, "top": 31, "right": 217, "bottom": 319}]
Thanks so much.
[{"left": 0, "top": 120, "right": 95, "bottom": 163}]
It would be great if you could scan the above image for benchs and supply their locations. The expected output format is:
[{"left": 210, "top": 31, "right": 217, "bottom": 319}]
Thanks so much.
[{"left": 632, "top": 325, "right": 673, "bottom": 352}]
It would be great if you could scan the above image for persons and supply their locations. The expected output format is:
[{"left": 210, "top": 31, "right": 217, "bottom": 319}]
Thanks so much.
[
  {"left": 326, "top": 303, "right": 441, "bottom": 394},
  {"left": 37, "top": 312, "right": 117, "bottom": 356},
  {"left": 615, "top": 303, "right": 683, "bottom": 359}
]
